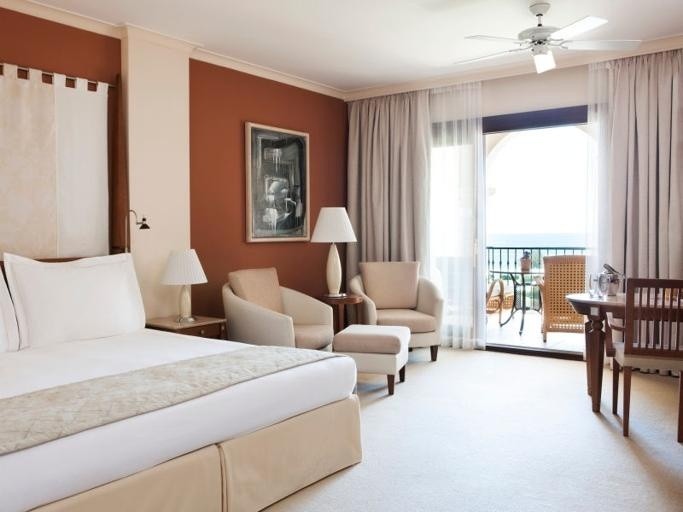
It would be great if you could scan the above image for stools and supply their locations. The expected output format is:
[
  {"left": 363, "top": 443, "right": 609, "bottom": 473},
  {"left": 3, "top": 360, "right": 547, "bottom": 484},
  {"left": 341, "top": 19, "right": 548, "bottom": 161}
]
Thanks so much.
[{"left": 329, "top": 322, "right": 409, "bottom": 395}]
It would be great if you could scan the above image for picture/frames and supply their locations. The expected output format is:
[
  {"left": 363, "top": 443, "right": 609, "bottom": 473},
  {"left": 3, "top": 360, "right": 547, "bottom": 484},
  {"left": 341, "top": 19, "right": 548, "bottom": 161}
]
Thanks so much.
[{"left": 242, "top": 120, "right": 311, "bottom": 245}]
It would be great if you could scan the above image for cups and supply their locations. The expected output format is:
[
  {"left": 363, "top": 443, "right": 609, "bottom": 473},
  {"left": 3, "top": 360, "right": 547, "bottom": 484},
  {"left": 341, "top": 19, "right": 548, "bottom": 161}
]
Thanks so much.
[
  {"left": 520, "top": 258, "right": 531, "bottom": 272},
  {"left": 587, "top": 271, "right": 597, "bottom": 298},
  {"left": 596, "top": 272, "right": 612, "bottom": 300}
]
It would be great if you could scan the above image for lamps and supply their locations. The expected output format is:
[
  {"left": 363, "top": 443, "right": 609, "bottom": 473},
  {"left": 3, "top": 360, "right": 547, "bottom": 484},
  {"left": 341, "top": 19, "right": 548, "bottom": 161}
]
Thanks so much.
[
  {"left": 155, "top": 249, "right": 209, "bottom": 324},
  {"left": 306, "top": 208, "right": 358, "bottom": 298},
  {"left": 123, "top": 208, "right": 150, "bottom": 251}
]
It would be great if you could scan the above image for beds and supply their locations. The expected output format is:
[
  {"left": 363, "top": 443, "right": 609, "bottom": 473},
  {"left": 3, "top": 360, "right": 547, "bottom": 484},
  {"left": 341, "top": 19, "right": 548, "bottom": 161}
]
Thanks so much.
[{"left": 0, "top": 256, "right": 364, "bottom": 511}]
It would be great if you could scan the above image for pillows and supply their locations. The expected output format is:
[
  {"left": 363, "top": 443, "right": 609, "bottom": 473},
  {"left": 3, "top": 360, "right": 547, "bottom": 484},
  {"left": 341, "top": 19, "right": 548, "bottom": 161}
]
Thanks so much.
[
  {"left": 1, "top": 264, "right": 20, "bottom": 353},
  {"left": 2, "top": 250, "right": 146, "bottom": 350}
]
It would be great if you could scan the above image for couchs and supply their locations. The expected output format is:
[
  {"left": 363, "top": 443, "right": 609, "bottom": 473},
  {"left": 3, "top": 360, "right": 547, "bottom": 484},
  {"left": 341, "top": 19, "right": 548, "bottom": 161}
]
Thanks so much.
[
  {"left": 347, "top": 259, "right": 444, "bottom": 364},
  {"left": 219, "top": 265, "right": 335, "bottom": 357}
]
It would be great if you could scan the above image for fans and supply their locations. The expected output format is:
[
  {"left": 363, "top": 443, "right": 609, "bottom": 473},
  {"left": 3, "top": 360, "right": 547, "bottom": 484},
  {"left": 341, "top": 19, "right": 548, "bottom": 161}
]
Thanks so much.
[{"left": 453, "top": 0, "right": 643, "bottom": 78}]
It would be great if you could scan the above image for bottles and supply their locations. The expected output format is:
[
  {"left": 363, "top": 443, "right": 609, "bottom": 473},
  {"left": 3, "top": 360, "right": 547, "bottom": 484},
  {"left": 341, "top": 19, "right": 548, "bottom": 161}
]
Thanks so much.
[{"left": 523, "top": 252, "right": 530, "bottom": 258}]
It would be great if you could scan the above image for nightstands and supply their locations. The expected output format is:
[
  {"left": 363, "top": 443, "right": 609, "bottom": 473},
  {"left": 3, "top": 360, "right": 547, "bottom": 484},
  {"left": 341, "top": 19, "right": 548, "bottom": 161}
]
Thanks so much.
[{"left": 146, "top": 314, "right": 228, "bottom": 342}]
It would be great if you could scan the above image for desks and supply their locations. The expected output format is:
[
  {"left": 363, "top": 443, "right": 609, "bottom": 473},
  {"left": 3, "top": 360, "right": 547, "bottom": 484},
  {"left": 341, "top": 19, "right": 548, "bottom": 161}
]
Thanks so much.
[
  {"left": 489, "top": 265, "right": 546, "bottom": 335},
  {"left": 564, "top": 289, "right": 682, "bottom": 416}
]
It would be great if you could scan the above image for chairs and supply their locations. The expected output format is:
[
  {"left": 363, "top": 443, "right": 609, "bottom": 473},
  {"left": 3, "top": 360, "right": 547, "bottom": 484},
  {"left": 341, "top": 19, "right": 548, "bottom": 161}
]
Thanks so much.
[
  {"left": 533, "top": 254, "right": 588, "bottom": 344},
  {"left": 612, "top": 277, "right": 683, "bottom": 446},
  {"left": 436, "top": 259, "right": 516, "bottom": 324}
]
